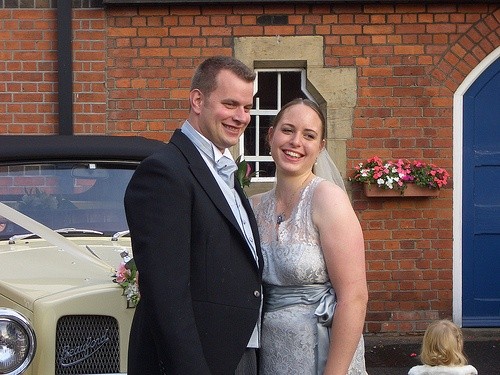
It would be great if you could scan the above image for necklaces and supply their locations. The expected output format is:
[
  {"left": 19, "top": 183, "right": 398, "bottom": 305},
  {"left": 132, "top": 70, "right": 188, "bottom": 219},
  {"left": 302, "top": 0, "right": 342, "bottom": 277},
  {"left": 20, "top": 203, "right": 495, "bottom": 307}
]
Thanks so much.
[{"left": 276, "top": 172, "right": 313, "bottom": 223}]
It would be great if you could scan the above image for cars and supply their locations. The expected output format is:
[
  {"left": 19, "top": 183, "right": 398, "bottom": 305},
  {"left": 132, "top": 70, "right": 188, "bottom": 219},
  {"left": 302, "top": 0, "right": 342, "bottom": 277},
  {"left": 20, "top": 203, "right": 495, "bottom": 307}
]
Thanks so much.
[{"left": 0, "top": 135, "right": 169, "bottom": 374}]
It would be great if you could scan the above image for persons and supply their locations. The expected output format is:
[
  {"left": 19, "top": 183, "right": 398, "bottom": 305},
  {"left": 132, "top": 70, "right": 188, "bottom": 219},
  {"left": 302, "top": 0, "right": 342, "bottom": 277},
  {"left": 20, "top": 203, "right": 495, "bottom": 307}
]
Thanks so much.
[
  {"left": 247, "top": 99, "right": 368, "bottom": 375},
  {"left": 124, "top": 55, "right": 265, "bottom": 375},
  {"left": 408, "top": 320, "right": 478, "bottom": 375}
]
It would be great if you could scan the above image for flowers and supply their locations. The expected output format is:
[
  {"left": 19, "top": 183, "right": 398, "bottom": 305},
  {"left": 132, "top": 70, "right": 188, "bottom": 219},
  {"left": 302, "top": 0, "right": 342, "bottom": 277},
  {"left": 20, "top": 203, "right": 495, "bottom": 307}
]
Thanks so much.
[
  {"left": 347, "top": 155, "right": 451, "bottom": 196},
  {"left": 111, "top": 257, "right": 141, "bottom": 308},
  {"left": 235, "top": 154, "right": 268, "bottom": 189}
]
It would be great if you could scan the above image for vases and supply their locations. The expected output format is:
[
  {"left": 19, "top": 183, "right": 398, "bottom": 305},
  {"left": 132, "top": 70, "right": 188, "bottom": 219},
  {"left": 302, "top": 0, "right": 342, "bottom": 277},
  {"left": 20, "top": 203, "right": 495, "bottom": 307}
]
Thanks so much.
[{"left": 364, "top": 183, "right": 440, "bottom": 196}]
